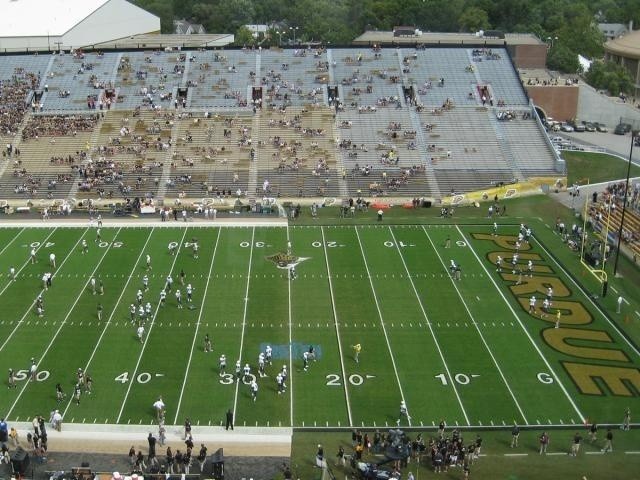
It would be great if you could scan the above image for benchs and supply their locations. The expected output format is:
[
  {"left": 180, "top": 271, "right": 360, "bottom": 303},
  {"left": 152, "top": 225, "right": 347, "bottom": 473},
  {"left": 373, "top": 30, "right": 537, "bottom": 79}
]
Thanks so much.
[
  {"left": 0, "top": 44, "right": 558, "bottom": 203},
  {"left": 588, "top": 180, "right": 639, "bottom": 258}
]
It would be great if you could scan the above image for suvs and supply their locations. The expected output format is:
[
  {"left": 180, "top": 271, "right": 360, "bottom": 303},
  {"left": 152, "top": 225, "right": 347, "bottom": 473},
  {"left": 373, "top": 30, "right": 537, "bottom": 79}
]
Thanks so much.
[{"left": 615, "top": 123, "right": 631, "bottom": 135}]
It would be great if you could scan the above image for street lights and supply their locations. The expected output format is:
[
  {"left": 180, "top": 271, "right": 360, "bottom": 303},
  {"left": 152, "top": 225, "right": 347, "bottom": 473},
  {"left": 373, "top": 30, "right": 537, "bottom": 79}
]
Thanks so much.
[
  {"left": 547, "top": 36, "right": 559, "bottom": 47},
  {"left": 613, "top": 130, "right": 640, "bottom": 275},
  {"left": 289, "top": 27, "right": 300, "bottom": 44},
  {"left": 276, "top": 31, "right": 286, "bottom": 45}
]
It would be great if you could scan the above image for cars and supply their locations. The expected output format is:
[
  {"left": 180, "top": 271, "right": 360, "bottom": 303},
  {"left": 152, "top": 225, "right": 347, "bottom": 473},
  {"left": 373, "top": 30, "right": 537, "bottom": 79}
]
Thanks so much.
[
  {"left": 540, "top": 116, "right": 585, "bottom": 151},
  {"left": 594, "top": 121, "right": 609, "bottom": 132},
  {"left": 582, "top": 120, "right": 596, "bottom": 132}
]
